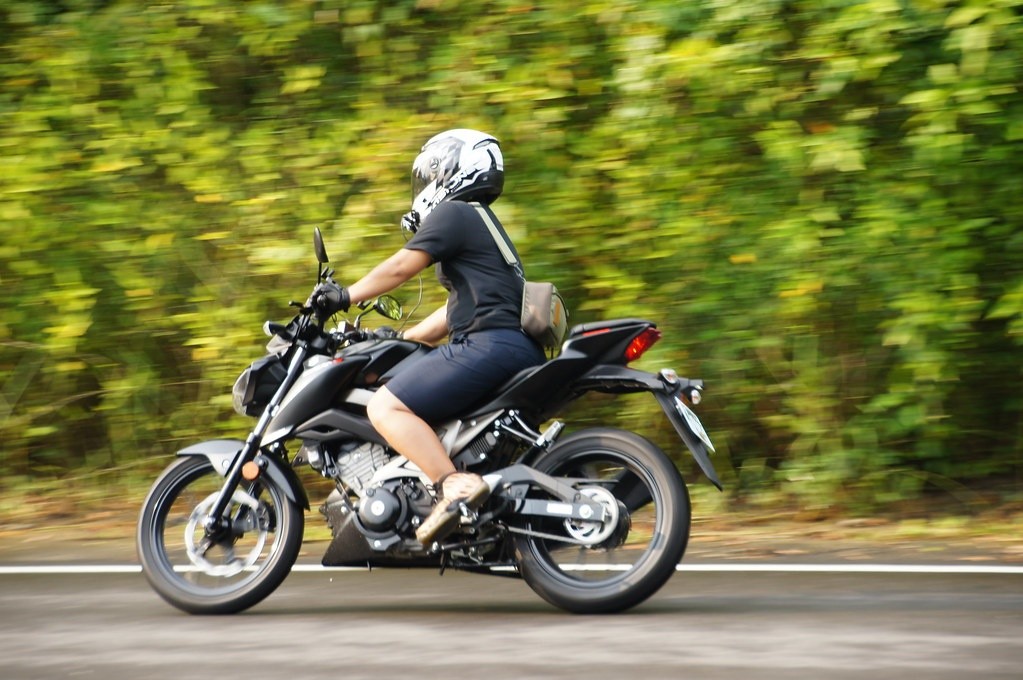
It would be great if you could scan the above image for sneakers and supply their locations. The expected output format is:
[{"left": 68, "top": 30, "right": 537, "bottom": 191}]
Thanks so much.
[{"left": 415, "top": 472, "right": 489, "bottom": 546}]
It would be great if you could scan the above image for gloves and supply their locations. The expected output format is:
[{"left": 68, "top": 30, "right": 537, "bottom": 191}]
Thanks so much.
[
  {"left": 310, "top": 283, "right": 350, "bottom": 320},
  {"left": 374, "top": 325, "right": 404, "bottom": 341}
]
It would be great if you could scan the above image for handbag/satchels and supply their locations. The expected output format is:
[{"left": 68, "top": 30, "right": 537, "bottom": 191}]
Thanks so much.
[{"left": 521, "top": 282, "right": 568, "bottom": 348}]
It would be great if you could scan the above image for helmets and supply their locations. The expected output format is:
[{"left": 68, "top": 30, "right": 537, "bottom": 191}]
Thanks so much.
[{"left": 400, "top": 128, "right": 504, "bottom": 234}]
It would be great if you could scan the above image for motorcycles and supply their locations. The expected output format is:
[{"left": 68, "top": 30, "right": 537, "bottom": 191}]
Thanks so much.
[{"left": 137, "top": 229, "right": 725, "bottom": 617}]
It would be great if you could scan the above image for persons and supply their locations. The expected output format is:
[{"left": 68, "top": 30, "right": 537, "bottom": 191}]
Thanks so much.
[{"left": 307, "top": 129, "right": 546, "bottom": 545}]
